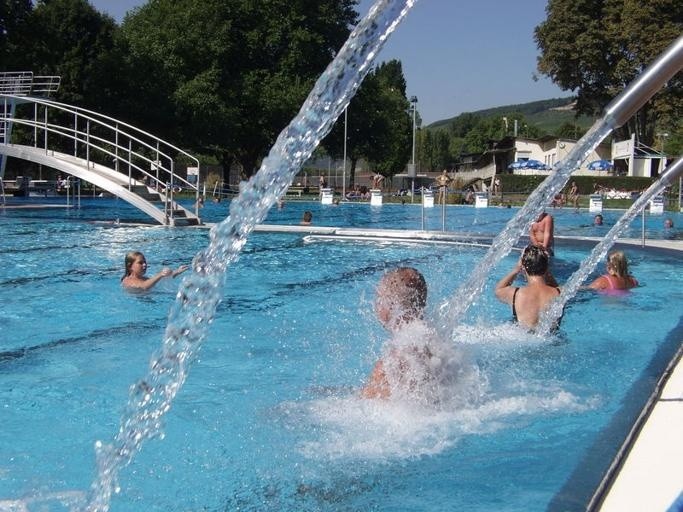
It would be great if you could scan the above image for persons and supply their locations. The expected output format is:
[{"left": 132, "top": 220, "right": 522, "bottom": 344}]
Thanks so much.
[
  {"left": 664, "top": 218, "right": 673, "bottom": 228},
  {"left": 568, "top": 181, "right": 580, "bottom": 211},
  {"left": 435, "top": 169, "right": 506, "bottom": 209},
  {"left": 119, "top": 251, "right": 189, "bottom": 290},
  {"left": 492, "top": 243, "right": 567, "bottom": 336},
  {"left": 319, "top": 169, "right": 385, "bottom": 205},
  {"left": 55, "top": 176, "right": 64, "bottom": 189},
  {"left": 588, "top": 250, "right": 638, "bottom": 295},
  {"left": 141, "top": 170, "right": 222, "bottom": 208},
  {"left": 594, "top": 215, "right": 604, "bottom": 225},
  {"left": 299, "top": 212, "right": 314, "bottom": 224},
  {"left": 359, "top": 267, "right": 445, "bottom": 401},
  {"left": 553, "top": 193, "right": 564, "bottom": 208},
  {"left": 527, "top": 205, "right": 556, "bottom": 257}
]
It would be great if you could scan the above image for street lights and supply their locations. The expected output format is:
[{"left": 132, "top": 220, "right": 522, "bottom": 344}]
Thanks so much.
[{"left": 410, "top": 95, "right": 418, "bottom": 203}]
